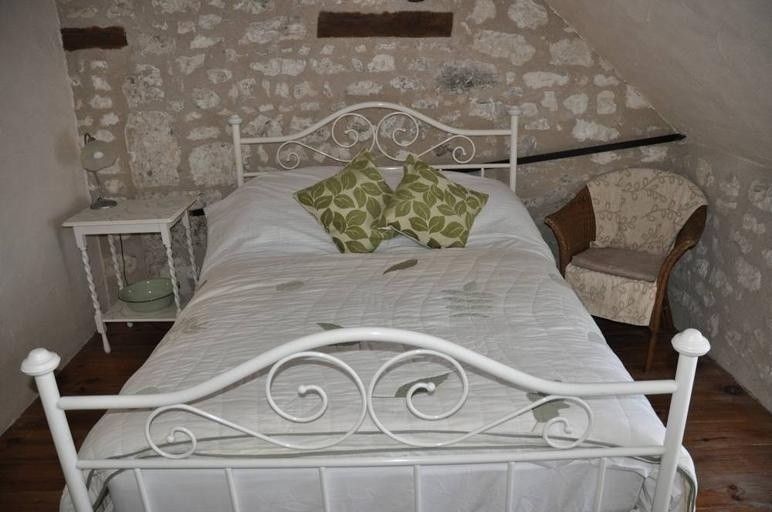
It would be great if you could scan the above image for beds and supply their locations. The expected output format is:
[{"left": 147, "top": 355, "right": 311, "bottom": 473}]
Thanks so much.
[{"left": 18, "top": 100, "right": 711, "bottom": 510}]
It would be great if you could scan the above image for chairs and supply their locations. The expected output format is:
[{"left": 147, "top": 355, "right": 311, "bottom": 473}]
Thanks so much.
[{"left": 544, "top": 164, "right": 709, "bottom": 375}]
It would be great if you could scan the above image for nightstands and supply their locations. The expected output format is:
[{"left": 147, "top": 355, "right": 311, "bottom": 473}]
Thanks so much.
[{"left": 62, "top": 193, "right": 207, "bottom": 353}]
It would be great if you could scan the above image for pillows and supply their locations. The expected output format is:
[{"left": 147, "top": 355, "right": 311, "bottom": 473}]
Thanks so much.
[{"left": 293, "top": 151, "right": 487, "bottom": 254}]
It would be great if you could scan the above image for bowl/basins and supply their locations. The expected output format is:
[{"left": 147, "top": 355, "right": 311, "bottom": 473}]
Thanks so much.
[{"left": 115, "top": 276, "right": 182, "bottom": 313}]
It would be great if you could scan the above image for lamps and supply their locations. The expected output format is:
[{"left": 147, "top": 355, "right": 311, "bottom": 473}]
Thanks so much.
[{"left": 76, "top": 131, "right": 120, "bottom": 208}]
